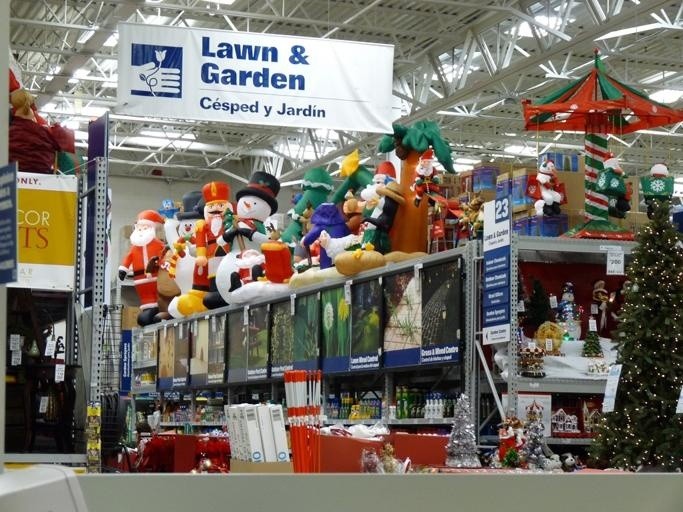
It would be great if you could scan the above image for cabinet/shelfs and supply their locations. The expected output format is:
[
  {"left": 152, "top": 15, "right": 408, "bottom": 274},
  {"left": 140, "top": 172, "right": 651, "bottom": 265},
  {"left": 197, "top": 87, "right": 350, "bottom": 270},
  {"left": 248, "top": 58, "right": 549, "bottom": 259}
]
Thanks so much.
[
  {"left": 473, "top": 233, "right": 641, "bottom": 467},
  {"left": 159, "top": 315, "right": 193, "bottom": 430},
  {"left": 132, "top": 322, "right": 160, "bottom": 446},
  {"left": 273, "top": 284, "right": 321, "bottom": 428},
  {"left": 322, "top": 264, "right": 385, "bottom": 428},
  {"left": 193, "top": 308, "right": 226, "bottom": 430},
  {"left": 386, "top": 242, "right": 475, "bottom": 431},
  {"left": 226, "top": 296, "right": 272, "bottom": 428}
]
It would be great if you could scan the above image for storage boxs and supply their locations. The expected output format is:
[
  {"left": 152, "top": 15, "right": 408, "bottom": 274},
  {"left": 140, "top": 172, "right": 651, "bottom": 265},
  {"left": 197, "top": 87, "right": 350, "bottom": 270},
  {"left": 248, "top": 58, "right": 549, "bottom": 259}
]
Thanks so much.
[{"left": 436, "top": 158, "right": 652, "bottom": 233}]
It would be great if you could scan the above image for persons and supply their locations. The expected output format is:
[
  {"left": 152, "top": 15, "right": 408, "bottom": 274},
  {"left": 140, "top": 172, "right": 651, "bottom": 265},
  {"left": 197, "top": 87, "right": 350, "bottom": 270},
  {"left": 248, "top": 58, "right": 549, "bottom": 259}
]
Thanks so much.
[
  {"left": 120, "top": 119, "right": 484, "bottom": 325},
  {"left": 521, "top": 279, "right": 617, "bottom": 378},
  {"left": 120, "top": 120, "right": 483, "bottom": 323}
]
